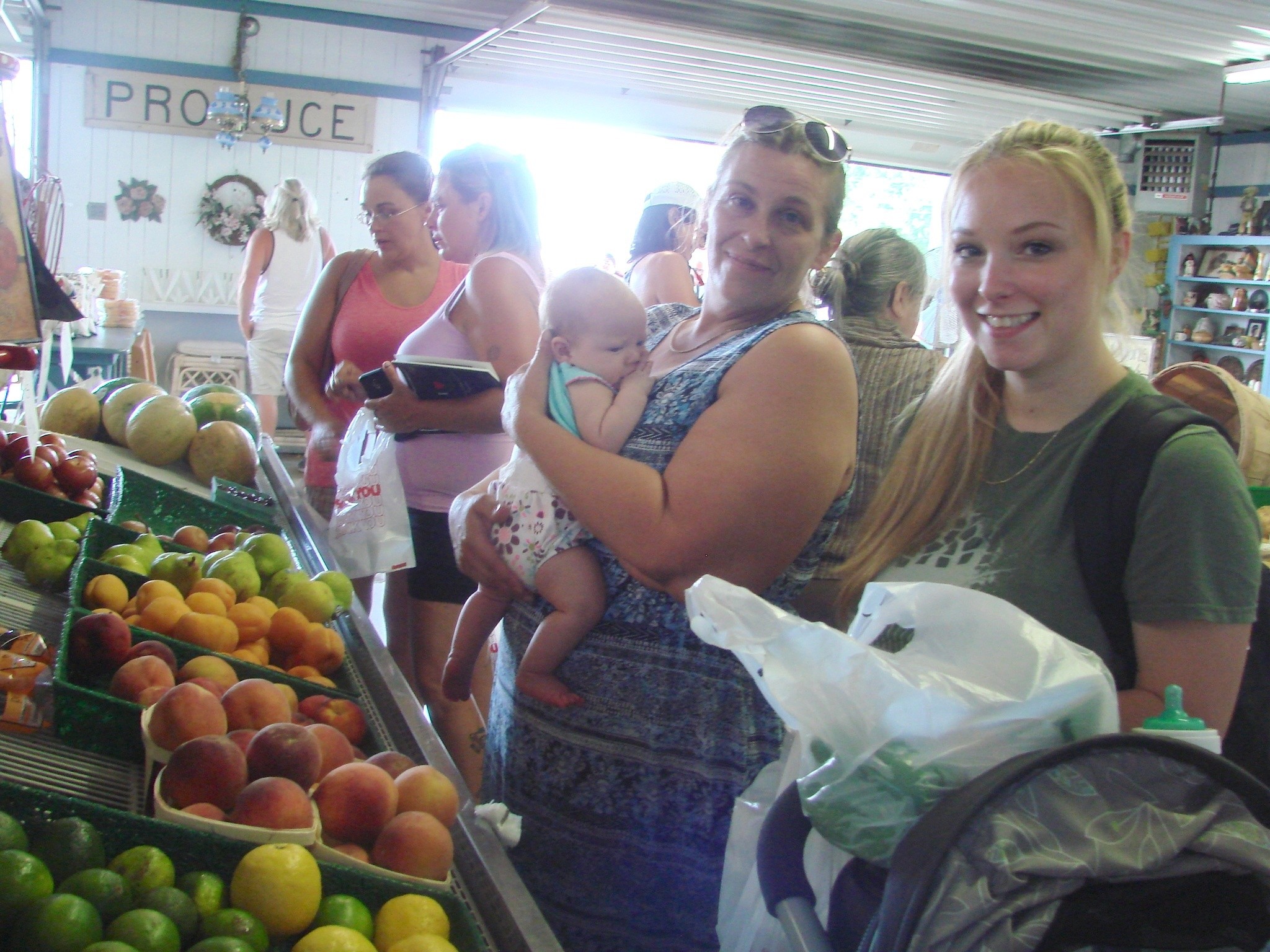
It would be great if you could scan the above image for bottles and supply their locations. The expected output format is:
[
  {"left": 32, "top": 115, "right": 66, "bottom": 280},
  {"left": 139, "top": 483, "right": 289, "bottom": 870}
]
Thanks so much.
[
  {"left": 1131, "top": 685, "right": 1223, "bottom": 756},
  {"left": 1183, "top": 291, "right": 1198, "bottom": 306},
  {"left": 1231, "top": 288, "right": 1246, "bottom": 311},
  {"left": 1176, "top": 218, "right": 1188, "bottom": 235}
]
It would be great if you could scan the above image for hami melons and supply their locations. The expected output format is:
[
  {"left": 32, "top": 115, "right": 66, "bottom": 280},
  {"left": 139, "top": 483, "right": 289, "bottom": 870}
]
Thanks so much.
[
  {"left": 179, "top": 384, "right": 253, "bottom": 410},
  {"left": 187, "top": 393, "right": 262, "bottom": 452},
  {"left": 92, "top": 377, "right": 145, "bottom": 405},
  {"left": 40, "top": 381, "right": 258, "bottom": 488}
]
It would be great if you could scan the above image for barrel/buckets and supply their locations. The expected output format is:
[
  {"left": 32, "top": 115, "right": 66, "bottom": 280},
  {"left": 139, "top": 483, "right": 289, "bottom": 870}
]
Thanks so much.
[{"left": 1148, "top": 362, "right": 1270, "bottom": 489}]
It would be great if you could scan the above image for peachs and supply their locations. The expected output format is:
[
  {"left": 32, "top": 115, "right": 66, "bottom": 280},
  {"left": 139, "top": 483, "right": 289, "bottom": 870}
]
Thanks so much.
[
  {"left": 65, "top": 575, "right": 459, "bottom": 881},
  {"left": 120, "top": 519, "right": 268, "bottom": 552}
]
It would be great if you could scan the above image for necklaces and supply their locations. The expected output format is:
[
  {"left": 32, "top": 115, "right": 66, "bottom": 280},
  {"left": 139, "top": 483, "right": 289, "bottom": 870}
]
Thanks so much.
[
  {"left": 669, "top": 296, "right": 802, "bottom": 353},
  {"left": 978, "top": 364, "right": 1123, "bottom": 485}
]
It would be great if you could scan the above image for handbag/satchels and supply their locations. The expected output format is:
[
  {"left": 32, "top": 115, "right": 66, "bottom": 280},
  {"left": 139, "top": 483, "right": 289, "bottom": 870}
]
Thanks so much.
[
  {"left": 686, "top": 573, "right": 1120, "bottom": 866},
  {"left": 717, "top": 730, "right": 854, "bottom": 952},
  {"left": 328, "top": 408, "right": 416, "bottom": 580}
]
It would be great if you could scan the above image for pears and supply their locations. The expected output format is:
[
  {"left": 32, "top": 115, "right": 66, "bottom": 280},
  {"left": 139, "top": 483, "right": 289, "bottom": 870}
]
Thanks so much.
[
  {"left": 99, "top": 530, "right": 355, "bottom": 625},
  {"left": 0, "top": 512, "right": 99, "bottom": 592}
]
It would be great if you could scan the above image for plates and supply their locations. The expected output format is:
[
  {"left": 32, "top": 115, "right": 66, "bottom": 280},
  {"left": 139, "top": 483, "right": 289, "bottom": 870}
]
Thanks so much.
[
  {"left": 1216, "top": 356, "right": 1244, "bottom": 380},
  {"left": 1245, "top": 358, "right": 1264, "bottom": 381}
]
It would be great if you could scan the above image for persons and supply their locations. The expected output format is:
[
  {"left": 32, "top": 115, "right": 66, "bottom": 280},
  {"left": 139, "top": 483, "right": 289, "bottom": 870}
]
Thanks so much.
[
  {"left": 776, "top": 223, "right": 949, "bottom": 634},
  {"left": 838, "top": 116, "right": 1262, "bottom": 777},
  {"left": 238, "top": 176, "right": 337, "bottom": 473},
  {"left": 437, "top": 268, "right": 656, "bottom": 708},
  {"left": 285, "top": 151, "right": 470, "bottom": 519},
  {"left": 362, "top": 141, "right": 541, "bottom": 792},
  {"left": 625, "top": 180, "right": 709, "bottom": 310},
  {"left": 446, "top": 101, "right": 859, "bottom": 952}
]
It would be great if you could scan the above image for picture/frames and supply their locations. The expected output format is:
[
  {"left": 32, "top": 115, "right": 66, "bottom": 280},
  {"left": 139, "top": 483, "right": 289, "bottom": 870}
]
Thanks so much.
[{"left": 1245, "top": 318, "right": 1267, "bottom": 339}]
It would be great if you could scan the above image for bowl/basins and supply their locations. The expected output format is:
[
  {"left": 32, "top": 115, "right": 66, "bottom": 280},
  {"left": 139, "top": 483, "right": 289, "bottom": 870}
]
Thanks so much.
[
  {"left": 1145, "top": 274, "right": 1165, "bottom": 287},
  {"left": 1148, "top": 220, "right": 1171, "bottom": 236},
  {"left": 1145, "top": 248, "right": 1169, "bottom": 263},
  {"left": 1208, "top": 293, "right": 1230, "bottom": 310}
]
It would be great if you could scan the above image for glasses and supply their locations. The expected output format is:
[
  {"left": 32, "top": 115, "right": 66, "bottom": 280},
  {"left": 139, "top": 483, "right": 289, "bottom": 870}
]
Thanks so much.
[
  {"left": 723, "top": 104, "right": 854, "bottom": 165},
  {"left": 357, "top": 204, "right": 417, "bottom": 225}
]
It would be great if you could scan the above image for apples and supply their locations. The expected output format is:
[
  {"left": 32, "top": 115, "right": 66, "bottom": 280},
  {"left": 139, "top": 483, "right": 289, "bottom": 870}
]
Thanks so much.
[{"left": 0, "top": 427, "right": 103, "bottom": 509}]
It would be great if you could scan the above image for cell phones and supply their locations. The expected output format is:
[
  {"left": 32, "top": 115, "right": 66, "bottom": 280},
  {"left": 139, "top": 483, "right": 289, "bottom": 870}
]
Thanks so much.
[{"left": 358, "top": 368, "right": 393, "bottom": 400}]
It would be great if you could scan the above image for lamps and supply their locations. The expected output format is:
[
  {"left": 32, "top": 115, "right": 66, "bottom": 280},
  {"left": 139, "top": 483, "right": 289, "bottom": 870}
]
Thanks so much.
[
  {"left": 208, "top": 73, "right": 286, "bottom": 159},
  {"left": 1220, "top": 57, "right": 1270, "bottom": 86}
]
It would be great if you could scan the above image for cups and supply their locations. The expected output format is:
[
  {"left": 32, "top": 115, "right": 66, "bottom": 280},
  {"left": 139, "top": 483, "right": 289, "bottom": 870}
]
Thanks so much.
[{"left": 1174, "top": 332, "right": 1187, "bottom": 340}]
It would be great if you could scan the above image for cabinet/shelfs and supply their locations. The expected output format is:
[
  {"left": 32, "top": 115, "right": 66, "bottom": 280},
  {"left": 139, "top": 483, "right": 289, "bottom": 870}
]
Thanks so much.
[{"left": 1154, "top": 233, "right": 1270, "bottom": 399}]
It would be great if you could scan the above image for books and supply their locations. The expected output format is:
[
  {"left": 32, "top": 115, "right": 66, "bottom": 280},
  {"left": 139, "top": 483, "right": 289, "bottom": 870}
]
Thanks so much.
[{"left": 393, "top": 352, "right": 503, "bottom": 401}]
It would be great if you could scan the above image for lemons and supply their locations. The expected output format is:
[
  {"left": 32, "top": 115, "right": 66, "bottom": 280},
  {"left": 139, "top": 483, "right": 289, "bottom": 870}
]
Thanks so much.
[{"left": 0, "top": 816, "right": 458, "bottom": 952}]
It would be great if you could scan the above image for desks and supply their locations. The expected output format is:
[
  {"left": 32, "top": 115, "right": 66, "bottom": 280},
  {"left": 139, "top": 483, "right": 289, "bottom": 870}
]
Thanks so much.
[{"left": 33, "top": 313, "right": 153, "bottom": 413}]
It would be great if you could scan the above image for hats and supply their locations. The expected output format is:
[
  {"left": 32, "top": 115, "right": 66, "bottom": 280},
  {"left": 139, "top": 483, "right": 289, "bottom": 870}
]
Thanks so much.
[{"left": 643, "top": 182, "right": 701, "bottom": 210}]
